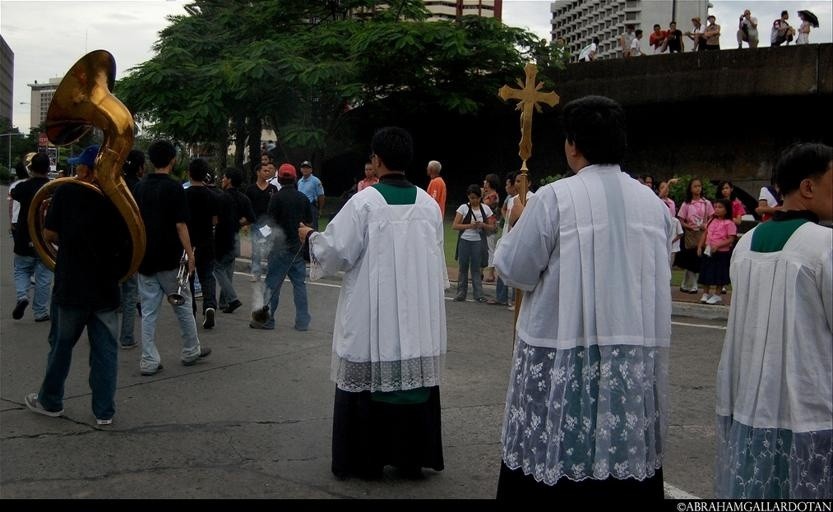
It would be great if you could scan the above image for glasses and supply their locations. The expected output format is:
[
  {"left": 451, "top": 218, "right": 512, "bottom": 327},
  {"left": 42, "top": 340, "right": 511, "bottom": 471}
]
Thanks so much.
[{"left": 369, "top": 154, "right": 375, "bottom": 160}]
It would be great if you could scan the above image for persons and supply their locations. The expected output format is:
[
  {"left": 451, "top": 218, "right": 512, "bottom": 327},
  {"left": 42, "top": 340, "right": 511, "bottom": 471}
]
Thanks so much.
[
  {"left": 656, "top": 178, "right": 681, "bottom": 217},
  {"left": 213, "top": 167, "right": 257, "bottom": 314},
  {"left": 644, "top": 173, "right": 655, "bottom": 190},
  {"left": 715, "top": 179, "right": 746, "bottom": 294},
  {"left": 487, "top": 170, "right": 534, "bottom": 311},
  {"left": 770, "top": 11, "right": 795, "bottom": 47},
  {"left": 298, "top": 126, "right": 450, "bottom": 481},
  {"left": 552, "top": 38, "right": 569, "bottom": 65},
  {"left": 697, "top": 199, "right": 737, "bottom": 305},
  {"left": 452, "top": 184, "right": 497, "bottom": 303},
  {"left": 537, "top": 39, "right": 549, "bottom": 66},
  {"left": 664, "top": 199, "right": 684, "bottom": 269},
  {"left": 183, "top": 159, "right": 219, "bottom": 329},
  {"left": 248, "top": 163, "right": 312, "bottom": 331},
  {"left": 677, "top": 178, "right": 715, "bottom": 294},
  {"left": 578, "top": 37, "right": 600, "bottom": 62},
  {"left": 713, "top": 142, "right": 833, "bottom": 499},
  {"left": 261, "top": 140, "right": 277, "bottom": 152},
  {"left": 261, "top": 154, "right": 282, "bottom": 190},
  {"left": 630, "top": 30, "right": 646, "bottom": 56},
  {"left": 298, "top": 160, "right": 325, "bottom": 232},
  {"left": 493, "top": 94, "right": 673, "bottom": 499},
  {"left": 650, "top": 24, "right": 668, "bottom": 55},
  {"left": 501, "top": 172, "right": 517, "bottom": 221},
  {"left": 737, "top": 10, "right": 759, "bottom": 49},
  {"left": 477, "top": 173, "right": 500, "bottom": 282},
  {"left": 12, "top": 153, "right": 54, "bottom": 322},
  {"left": 621, "top": 25, "right": 636, "bottom": 58},
  {"left": 243, "top": 164, "right": 279, "bottom": 282},
  {"left": 24, "top": 144, "right": 134, "bottom": 426},
  {"left": 426, "top": 160, "right": 447, "bottom": 218},
  {"left": 796, "top": 14, "right": 811, "bottom": 45},
  {"left": 357, "top": 161, "right": 379, "bottom": 191},
  {"left": 667, "top": 22, "right": 685, "bottom": 53},
  {"left": 685, "top": 16, "right": 704, "bottom": 51},
  {"left": 705, "top": 16, "right": 721, "bottom": 51},
  {"left": 265, "top": 164, "right": 283, "bottom": 191},
  {"left": 119, "top": 150, "right": 146, "bottom": 350},
  {"left": 133, "top": 140, "right": 212, "bottom": 376},
  {"left": 8, "top": 163, "right": 38, "bottom": 285},
  {"left": 755, "top": 174, "right": 784, "bottom": 222}
]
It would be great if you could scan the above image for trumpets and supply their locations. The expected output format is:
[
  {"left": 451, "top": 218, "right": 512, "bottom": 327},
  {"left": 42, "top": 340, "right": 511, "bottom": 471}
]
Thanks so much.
[{"left": 167, "top": 246, "right": 197, "bottom": 306}]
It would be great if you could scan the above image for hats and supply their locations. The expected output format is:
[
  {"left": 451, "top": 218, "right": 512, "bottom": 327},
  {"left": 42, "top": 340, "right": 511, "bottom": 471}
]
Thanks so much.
[
  {"left": 27, "top": 153, "right": 51, "bottom": 174},
  {"left": 67, "top": 143, "right": 100, "bottom": 166},
  {"left": 278, "top": 163, "right": 296, "bottom": 179},
  {"left": 123, "top": 150, "right": 144, "bottom": 174},
  {"left": 300, "top": 161, "right": 312, "bottom": 168}
]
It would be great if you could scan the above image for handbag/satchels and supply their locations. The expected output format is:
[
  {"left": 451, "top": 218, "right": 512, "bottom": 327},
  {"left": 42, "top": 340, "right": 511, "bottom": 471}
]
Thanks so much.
[
  {"left": 684, "top": 199, "right": 706, "bottom": 249},
  {"left": 466, "top": 203, "right": 488, "bottom": 267}
]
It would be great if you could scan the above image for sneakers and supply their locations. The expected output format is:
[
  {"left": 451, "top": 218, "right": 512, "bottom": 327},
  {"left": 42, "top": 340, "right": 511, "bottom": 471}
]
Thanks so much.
[
  {"left": 35, "top": 314, "right": 50, "bottom": 321},
  {"left": 219, "top": 303, "right": 226, "bottom": 308},
  {"left": 121, "top": 341, "right": 138, "bottom": 349},
  {"left": 96, "top": 418, "right": 112, "bottom": 425},
  {"left": 202, "top": 307, "right": 215, "bottom": 328},
  {"left": 689, "top": 287, "right": 697, "bottom": 294},
  {"left": 223, "top": 300, "right": 241, "bottom": 313},
  {"left": 12, "top": 296, "right": 29, "bottom": 319},
  {"left": 25, "top": 392, "right": 64, "bottom": 417},
  {"left": 477, "top": 297, "right": 486, "bottom": 302},
  {"left": 680, "top": 284, "right": 688, "bottom": 292},
  {"left": 700, "top": 293, "right": 710, "bottom": 302},
  {"left": 453, "top": 296, "right": 465, "bottom": 302},
  {"left": 722, "top": 286, "right": 726, "bottom": 294},
  {"left": 706, "top": 293, "right": 722, "bottom": 304}
]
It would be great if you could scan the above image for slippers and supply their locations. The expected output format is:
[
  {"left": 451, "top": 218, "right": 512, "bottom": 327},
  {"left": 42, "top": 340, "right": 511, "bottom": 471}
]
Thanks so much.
[{"left": 487, "top": 299, "right": 505, "bottom": 305}]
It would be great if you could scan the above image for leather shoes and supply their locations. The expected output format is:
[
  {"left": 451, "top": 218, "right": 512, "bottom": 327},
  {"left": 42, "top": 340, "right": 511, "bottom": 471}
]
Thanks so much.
[{"left": 184, "top": 348, "right": 211, "bottom": 365}]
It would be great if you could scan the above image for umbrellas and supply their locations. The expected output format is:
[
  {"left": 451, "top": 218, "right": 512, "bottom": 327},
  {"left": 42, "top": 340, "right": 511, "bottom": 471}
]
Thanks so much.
[{"left": 797, "top": 10, "right": 819, "bottom": 28}]
